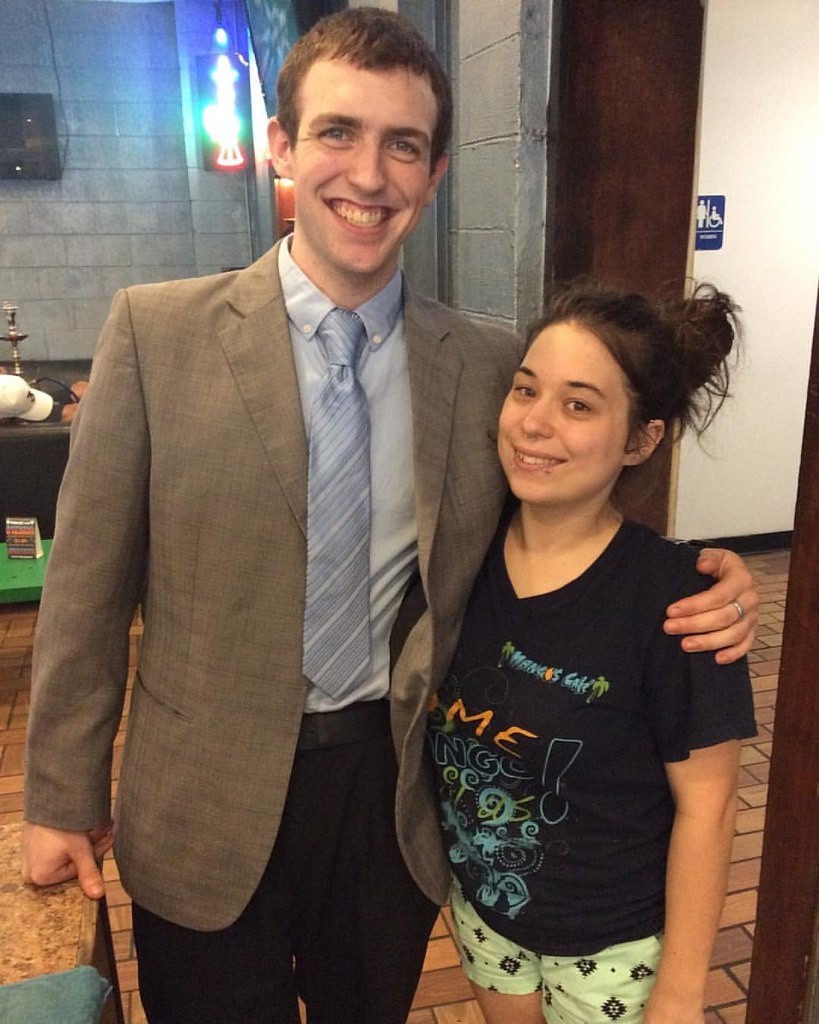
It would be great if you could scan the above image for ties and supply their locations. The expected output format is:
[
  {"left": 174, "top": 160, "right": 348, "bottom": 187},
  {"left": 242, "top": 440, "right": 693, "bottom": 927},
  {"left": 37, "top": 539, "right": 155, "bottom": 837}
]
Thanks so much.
[{"left": 302, "top": 309, "right": 371, "bottom": 701}]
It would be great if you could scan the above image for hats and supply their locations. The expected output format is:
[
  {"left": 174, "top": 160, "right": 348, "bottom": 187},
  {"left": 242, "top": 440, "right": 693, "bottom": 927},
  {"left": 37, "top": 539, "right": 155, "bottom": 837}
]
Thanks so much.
[{"left": 0, "top": 375, "right": 53, "bottom": 421}]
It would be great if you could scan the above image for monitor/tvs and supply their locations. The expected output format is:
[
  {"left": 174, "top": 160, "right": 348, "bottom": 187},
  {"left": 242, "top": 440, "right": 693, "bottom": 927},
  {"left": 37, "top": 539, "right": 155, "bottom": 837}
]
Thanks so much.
[{"left": 0, "top": 92, "right": 63, "bottom": 181}]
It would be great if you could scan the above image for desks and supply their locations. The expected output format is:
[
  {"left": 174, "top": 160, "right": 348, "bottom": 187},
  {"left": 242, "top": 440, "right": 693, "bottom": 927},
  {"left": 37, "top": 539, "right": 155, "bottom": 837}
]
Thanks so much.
[{"left": 0, "top": 823, "right": 125, "bottom": 1024}]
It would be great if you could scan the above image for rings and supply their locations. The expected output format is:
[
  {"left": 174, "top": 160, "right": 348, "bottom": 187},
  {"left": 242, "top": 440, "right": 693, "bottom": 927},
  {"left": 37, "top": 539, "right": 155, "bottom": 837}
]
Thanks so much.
[{"left": 732, "top": 599, "right": 746, "bottom": 619}]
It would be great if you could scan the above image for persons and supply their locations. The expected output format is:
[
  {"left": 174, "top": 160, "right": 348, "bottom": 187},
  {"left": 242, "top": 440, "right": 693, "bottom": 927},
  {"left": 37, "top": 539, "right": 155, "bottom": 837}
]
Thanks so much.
[
  {"left": 21, "top": 8, "right": 761, "bottom": 1024},
  {"left": 423, "top": 274, "right": 759, "bottom": 1024},
  {"left": 0, "top": 367, "right": 91, "bottom": 424}
]
